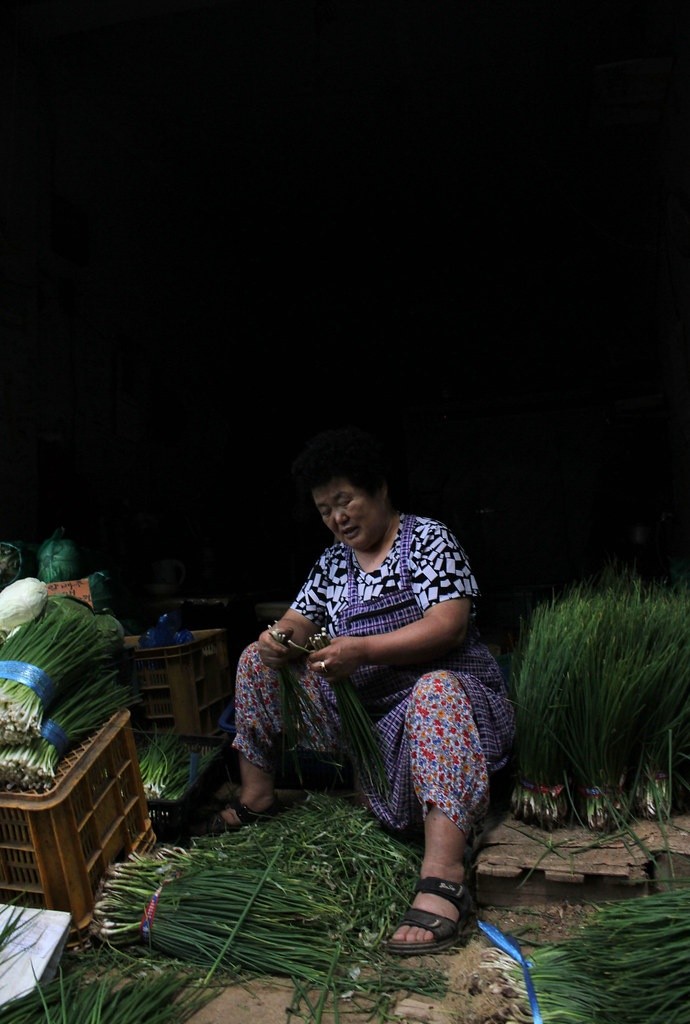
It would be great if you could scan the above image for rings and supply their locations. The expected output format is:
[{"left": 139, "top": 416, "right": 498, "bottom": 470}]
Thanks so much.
[{"left": 319, "top": 659, "right": 328, "bottom": 673}]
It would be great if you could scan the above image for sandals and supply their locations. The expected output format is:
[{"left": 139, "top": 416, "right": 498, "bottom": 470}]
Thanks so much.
[
  {"left": 386, "top": 875, "right": 478, "bottom": 955},
  {"left": 189, "top": 791, "right": 284, "bottom": 837}
]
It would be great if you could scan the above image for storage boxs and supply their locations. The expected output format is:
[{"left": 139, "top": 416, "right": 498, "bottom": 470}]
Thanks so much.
[
  {"left": 132, "top": 731, "right": 237, "bottom": 852},
  {"left": 219, "top": 699, "right": 347, "bottom": 791},
  {"left": 0, "top": 704, "right": 159, "bottom": 953},
  {"left": 119, "top": 627, "right": 233, "bottom": 736}
]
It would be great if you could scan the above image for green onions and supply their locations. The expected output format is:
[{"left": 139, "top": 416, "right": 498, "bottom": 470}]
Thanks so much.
[{"left": 2, "top": 587, "right": 690, "bottom": 1024}]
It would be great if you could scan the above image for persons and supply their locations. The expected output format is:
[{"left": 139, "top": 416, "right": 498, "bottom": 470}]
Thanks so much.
[{"left": 208, "top": 426, "right": 521, "bottom": 960}]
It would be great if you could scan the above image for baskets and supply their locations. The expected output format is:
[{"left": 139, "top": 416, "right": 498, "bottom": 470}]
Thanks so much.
[
  {"left": 120, "top": 627, "right": 233, "bottom": 734},
  {"left": 133, "top": 730, "right": 231, "bottom": 845},
  {"left": 0, "top": 708, "right": 156, "bottom": 954}
]
